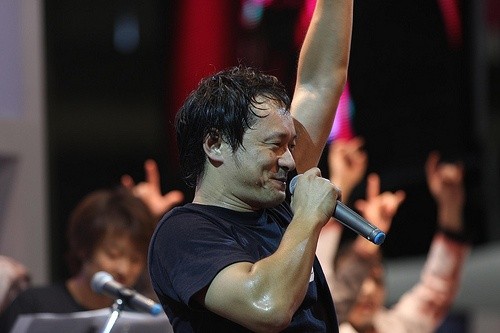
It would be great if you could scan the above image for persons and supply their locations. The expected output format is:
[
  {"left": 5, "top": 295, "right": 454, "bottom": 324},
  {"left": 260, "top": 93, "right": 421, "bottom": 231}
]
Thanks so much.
[
  {"left": 312, "top": 134, "right": 466, "bottom": 333},
  {"left": 148, "top": 0, "right": 355, "bottom": 333},
  {"left": 0, "top": 188, "right": 158, "bottom": 333},
  {"left": 0, "top": 159, "right": 183, "bottom": 315}
]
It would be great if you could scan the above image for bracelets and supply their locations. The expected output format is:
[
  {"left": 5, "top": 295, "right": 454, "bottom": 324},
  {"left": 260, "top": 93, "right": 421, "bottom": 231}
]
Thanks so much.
[{"left": 435, "top": 226, "right": 466, "bottom": 242}]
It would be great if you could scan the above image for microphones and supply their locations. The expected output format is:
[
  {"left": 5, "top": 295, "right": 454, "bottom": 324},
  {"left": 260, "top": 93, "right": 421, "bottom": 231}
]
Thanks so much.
[
  {"left": 289, "top": 174, "right": 385, "bottom": 245},
  {"left": 90, "top": 271, "right": 163, "bottom": 316}
]
